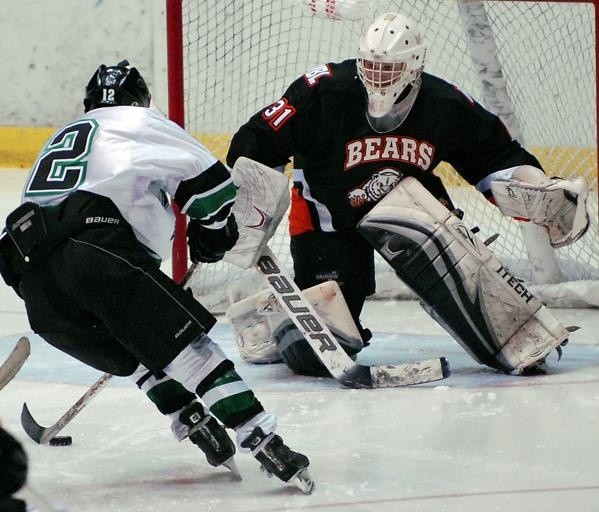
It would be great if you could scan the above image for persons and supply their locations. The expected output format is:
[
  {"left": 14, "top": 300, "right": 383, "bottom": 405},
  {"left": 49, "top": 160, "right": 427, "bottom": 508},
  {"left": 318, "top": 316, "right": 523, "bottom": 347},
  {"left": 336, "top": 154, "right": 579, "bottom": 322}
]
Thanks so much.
[
  {"left": 18, "top": 62, "right": 311, "bottom": 486},
  {"left": 220, "top": 12, "right": 592, "bottom": 379}
]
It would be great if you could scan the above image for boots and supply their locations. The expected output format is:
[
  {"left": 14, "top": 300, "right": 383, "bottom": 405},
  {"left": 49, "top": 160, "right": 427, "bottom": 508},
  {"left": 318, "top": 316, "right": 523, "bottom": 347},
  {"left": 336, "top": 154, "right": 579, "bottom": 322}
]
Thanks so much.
[
  {"left": 178, "top": 401, "right": 236, "bottom": 467},
  {"left": 240, "top": 426, "right": 310, "bottom": 483}
]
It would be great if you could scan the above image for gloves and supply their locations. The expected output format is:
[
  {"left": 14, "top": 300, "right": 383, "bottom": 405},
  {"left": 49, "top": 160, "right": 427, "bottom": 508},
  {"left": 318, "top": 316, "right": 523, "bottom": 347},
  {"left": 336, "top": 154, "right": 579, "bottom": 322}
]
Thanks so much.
[{"left": 185, "top": 213, "right": 240, "bottom": 264}]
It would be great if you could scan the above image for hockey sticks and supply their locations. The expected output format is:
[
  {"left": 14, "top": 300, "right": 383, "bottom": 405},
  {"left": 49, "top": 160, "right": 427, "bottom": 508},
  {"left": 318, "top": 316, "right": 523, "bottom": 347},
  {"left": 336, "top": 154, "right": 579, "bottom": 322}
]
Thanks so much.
[
  {"left": 22, "top": 261, "right": 206, "bottom": 445},
  {"left": 255, "top": 245, "right": 451, "bottom": 388},
  {"left": 1, "top": 335, "right": 30, "bottom": 392}
]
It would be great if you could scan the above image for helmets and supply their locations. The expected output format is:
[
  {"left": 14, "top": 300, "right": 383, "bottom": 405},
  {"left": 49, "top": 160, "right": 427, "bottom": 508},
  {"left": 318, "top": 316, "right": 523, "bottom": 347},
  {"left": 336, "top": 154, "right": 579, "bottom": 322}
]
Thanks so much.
[
  {"left": 84, "top": 63, "right": 151, "bottom": 114},
  {"left": 357, "top": 11, "right": 427, "bottom": 134}
]
